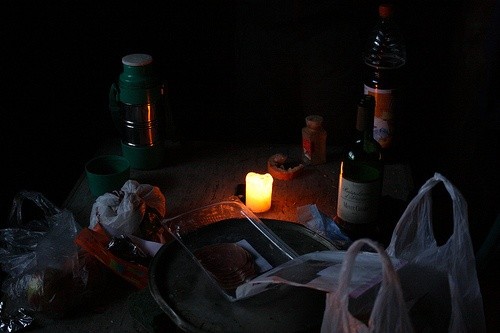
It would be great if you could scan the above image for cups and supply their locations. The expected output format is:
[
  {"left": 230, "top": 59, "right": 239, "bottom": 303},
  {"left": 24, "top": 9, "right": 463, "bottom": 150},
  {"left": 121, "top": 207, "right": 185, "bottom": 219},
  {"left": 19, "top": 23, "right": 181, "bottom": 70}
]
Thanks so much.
[{"left": 85, "top": 156, "right": 131, "bottom": 196}]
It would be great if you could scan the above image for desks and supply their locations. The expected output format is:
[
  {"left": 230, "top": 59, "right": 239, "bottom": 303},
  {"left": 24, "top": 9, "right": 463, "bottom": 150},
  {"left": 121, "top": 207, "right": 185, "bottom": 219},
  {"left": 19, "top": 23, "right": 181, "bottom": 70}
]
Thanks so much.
[{"left": 0, "top": 124, "right": 423, "bottom": 333}]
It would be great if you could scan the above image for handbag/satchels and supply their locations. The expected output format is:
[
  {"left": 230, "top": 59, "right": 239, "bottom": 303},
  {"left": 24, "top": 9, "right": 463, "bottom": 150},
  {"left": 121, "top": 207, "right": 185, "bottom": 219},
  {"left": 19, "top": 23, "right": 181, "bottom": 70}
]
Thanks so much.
[
  {"left": 0, "top": 192, "right": 94, "bottom": 313},
  {"left": 320, "top": 172, "right": 486, "bottom": 333}
]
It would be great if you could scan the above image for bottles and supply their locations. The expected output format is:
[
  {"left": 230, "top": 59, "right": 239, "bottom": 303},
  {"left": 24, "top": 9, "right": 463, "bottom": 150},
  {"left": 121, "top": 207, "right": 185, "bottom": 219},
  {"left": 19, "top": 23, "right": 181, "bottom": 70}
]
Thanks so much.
[
  {"left": 356, "top": 5, "right": 407, "bottom": 153},
  {"left": 336, "top": 94, "right": 385, "bottom": 226},
  {"left": 109, "top": 52, "right": 176, "bottom": 169},
  {"left": 302, "top": 115, "right": 328, "bottom": 164}
]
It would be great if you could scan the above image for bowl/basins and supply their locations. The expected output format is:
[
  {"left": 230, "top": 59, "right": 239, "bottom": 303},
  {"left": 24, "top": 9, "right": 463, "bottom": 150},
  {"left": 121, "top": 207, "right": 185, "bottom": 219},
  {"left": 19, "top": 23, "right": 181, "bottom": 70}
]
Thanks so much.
[{"left": 158, "top": 194, "right": 300, "bottom": 302}]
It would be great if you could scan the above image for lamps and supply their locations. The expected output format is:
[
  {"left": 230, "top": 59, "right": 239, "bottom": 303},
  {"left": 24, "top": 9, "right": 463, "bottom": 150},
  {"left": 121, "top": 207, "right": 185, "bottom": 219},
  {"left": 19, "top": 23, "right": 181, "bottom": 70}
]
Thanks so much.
[{"left": 245, "top": 171, "right": 275, "bottom": 214}]
